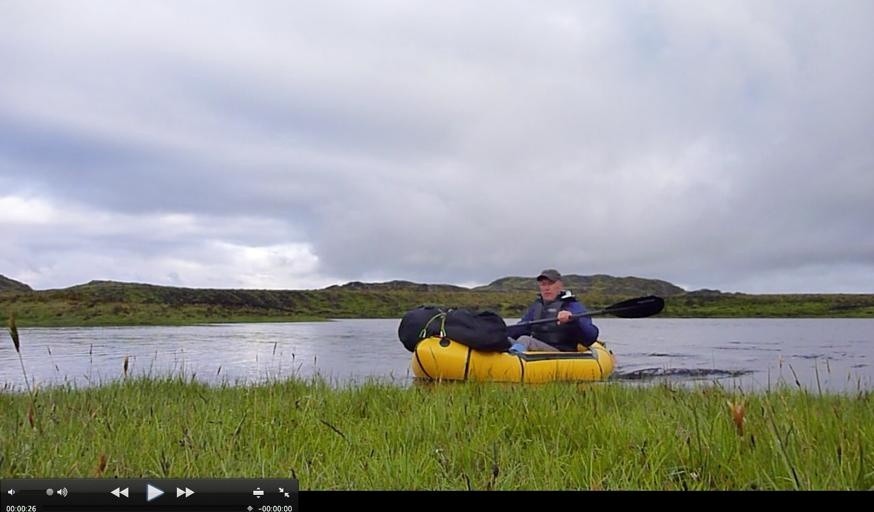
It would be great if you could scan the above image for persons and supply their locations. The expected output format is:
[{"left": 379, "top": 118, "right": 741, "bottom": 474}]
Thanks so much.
[{"left": 507, "top": 269, "right": 601, "bottom": 354}]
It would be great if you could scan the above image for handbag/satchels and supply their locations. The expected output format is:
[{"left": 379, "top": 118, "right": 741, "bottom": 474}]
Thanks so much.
[{"left": 398, "top": 305, "right": 509, "bottom": 351}]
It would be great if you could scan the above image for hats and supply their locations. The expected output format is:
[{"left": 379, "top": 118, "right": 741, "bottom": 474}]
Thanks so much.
[{"left": 537, "top": 269, "right": 561, "bottom": 282}]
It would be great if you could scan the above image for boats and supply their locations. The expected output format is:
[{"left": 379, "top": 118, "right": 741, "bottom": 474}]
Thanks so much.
[{"left": 409, "top": 335, "right": 618, "bottom": 385}]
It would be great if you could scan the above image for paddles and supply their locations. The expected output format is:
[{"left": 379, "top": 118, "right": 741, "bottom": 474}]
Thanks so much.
[{"left": 507, "top": 296, "right": 665, "bottom": 328}]
